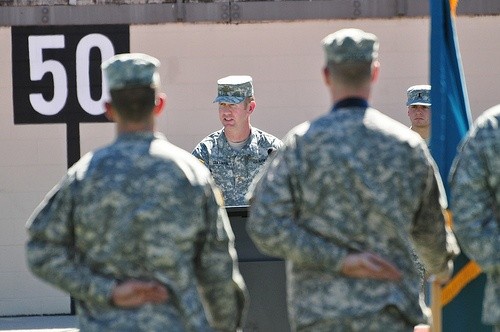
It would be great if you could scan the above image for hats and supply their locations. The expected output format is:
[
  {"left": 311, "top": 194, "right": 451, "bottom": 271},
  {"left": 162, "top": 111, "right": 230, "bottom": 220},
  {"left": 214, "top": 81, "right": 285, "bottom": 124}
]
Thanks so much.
[
  {"left": 321, "top": 29, "right": 377, "bottom": 64},
  {"left": 102, "top": 53, "right": 160, "bottom": 89},
  {"left": 406, "top": 85, "right": 431, "bottom": 106},
  {"left": 212, "top": 76, "right": 254, "bottom": 104}
]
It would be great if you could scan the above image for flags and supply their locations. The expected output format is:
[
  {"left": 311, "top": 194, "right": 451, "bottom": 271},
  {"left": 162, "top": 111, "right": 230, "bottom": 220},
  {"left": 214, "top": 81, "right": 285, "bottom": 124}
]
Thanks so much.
[{"left": 428, "top": 0, "right": 493, "bottom": 332}]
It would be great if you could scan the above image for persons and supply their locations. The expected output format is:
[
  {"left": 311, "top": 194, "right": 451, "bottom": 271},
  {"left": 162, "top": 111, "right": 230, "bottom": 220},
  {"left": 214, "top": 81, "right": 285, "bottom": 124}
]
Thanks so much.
[
  {"left": 191, "top": 74, "right": 283, "bottom": 205},
  {"left": 446, "top": 103, "right": 500, "bottom": 332},
  {"left": 245, "top": 27, "right": 459, "bottom": 332},
  {"left": 405, "top": 85, "right": 434, "bottom": 147},
  {"left": 24, "top": 52, "right": 250, "bottom": 332}
]
